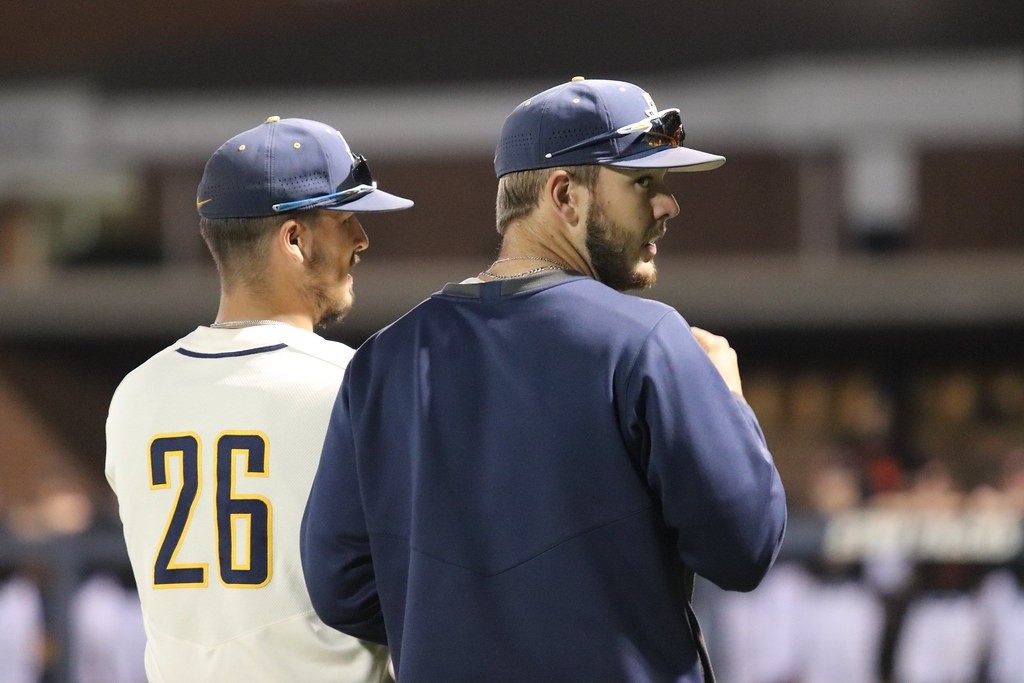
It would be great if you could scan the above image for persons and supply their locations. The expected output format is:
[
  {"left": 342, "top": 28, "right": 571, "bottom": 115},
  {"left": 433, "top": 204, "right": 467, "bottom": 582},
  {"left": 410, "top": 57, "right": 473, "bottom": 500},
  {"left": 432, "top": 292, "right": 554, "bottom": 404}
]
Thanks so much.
[
  {"left": 300, "top": 75, "right": 786, "bottom": 683},
  {"left": 103, "top": 119, "right": 372, "bottom": 680}
]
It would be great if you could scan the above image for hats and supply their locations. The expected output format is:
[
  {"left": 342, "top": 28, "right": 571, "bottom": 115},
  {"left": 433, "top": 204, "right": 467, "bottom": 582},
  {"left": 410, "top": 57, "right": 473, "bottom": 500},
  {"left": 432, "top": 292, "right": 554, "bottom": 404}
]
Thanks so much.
[
  {"left": 196, "top": 115, "right": 413, "bottom": 219},
  {"left": 495, "top": 76, "right": 726, "bottom": 178}
]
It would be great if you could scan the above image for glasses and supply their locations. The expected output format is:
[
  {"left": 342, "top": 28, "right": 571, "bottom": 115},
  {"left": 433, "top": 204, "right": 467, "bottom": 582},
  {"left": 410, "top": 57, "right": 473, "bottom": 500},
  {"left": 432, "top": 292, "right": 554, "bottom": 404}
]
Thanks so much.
[
  {"left": 271, "top": 152, "right": 376, "bottom": 213},
  {"left": 546, "top": 108, "right": 685, "bottom": 159}
]
